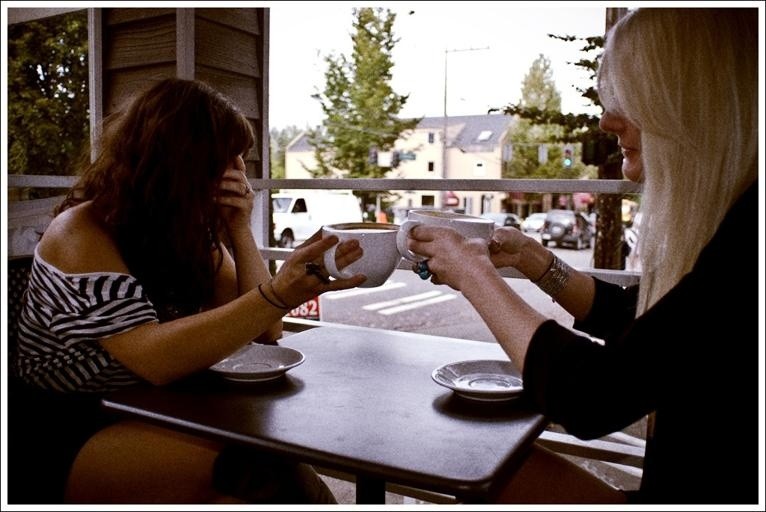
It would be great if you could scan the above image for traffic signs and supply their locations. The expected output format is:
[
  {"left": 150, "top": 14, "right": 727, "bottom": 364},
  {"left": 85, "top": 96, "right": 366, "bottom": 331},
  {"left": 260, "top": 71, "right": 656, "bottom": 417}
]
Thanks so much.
[{"left": 400, "top": 153, "right": 416, "bottom": 160}]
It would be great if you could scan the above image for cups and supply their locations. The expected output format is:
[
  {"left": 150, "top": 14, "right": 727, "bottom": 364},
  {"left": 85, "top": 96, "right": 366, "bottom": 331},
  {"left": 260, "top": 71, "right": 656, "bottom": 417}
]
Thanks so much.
[
  {"left": 395, "top": 209, "right": 497, "bottom": 270},
  {"left": 321, "top": 221, "right": 402, "bottom": 289}
]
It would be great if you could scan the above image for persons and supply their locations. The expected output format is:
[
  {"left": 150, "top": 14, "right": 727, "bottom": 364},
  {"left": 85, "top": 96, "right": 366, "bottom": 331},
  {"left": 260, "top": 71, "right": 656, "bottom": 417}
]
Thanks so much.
[
  {"left": 10, "top": 77, "right": 367, "bottom": 504},
  {"left": 406, "top": 8, "right": 759, "bottom": 504}
]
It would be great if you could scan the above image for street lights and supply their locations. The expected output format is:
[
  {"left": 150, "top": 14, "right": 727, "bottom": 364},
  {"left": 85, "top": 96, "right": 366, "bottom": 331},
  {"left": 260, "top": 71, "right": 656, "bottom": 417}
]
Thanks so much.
[
  {"left": 438, "top": 130, "right": 468, "bottom": 211},
  {"left": 369, "top": 144, "right": 377, "bottom": 164},
  {"left": 392, "top": 151, "right": 401, "bottom": 168}
]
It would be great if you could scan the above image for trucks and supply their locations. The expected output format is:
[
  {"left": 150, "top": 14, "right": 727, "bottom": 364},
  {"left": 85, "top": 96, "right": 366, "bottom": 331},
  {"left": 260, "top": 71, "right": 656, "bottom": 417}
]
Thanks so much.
[{"left": 271, "top": 189, "right": 365, "bottom": 249}]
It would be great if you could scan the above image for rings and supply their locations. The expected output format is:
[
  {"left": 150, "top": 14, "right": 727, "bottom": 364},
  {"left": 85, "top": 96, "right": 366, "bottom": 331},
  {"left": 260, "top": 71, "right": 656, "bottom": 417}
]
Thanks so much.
[
  {"left": 241, "top": 184, "right": 250, "bottom": 197},
  {"left": 304, "top": 262, "right": 330, "bottom": 285},
  {"left": 416, "top": 258, "right": 436, "bottom": 280}
]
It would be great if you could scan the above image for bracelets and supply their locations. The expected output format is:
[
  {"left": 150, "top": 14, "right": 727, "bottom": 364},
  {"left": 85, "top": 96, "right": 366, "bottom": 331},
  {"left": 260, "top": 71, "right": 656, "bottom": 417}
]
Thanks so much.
[
  {"left": 531, "top": 251, "right": 570, "bottom": 297},
  {"left": 258, "top": 277, "right": 297, "bottom": 310}
]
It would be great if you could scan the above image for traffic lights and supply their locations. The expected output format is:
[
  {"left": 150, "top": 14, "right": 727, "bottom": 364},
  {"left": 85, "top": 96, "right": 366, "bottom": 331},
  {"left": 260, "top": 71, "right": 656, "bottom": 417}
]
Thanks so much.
[{"left": 562, "top": 144, "right": 573, "bottom": 169}]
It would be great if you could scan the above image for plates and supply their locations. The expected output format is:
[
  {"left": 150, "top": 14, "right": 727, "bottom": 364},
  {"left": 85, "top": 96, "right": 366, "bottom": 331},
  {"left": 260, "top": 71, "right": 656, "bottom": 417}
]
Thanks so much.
[
  {"left": 430, "top": 359, "right": 520, "bottom": 404},
  {"left": 231, "top": 373, "right": 305, "bottom": 401},
  {"left": 210, "top": 343, "right": 305, "bottom": 383},
  {"left": 429, "top": 393, "right": 542, "bottom": 425}
]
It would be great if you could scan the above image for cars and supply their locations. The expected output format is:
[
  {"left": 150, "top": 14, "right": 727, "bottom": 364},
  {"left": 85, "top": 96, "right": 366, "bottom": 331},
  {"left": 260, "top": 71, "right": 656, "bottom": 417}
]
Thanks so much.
[{"left": 479, "top": 198, "right": 639, "bottom": 251}]
